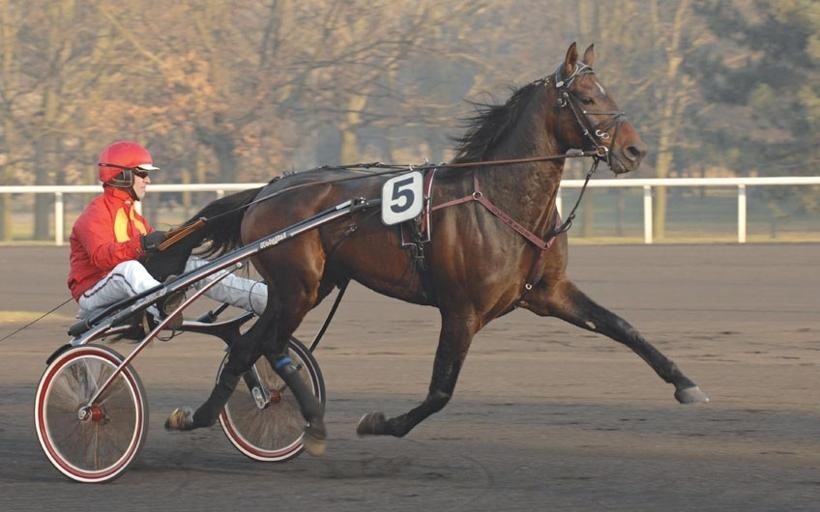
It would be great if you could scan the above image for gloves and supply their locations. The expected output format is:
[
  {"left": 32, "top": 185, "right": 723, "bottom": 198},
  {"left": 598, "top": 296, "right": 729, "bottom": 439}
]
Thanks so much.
[{"left": 140, "top": 230, "right": 168, "bottom": 251}]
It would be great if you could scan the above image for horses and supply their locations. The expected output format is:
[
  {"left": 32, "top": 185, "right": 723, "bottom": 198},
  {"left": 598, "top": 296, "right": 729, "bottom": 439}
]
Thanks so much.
[{"left": 108, "top": 43, "right": 710, "bottom": 438}]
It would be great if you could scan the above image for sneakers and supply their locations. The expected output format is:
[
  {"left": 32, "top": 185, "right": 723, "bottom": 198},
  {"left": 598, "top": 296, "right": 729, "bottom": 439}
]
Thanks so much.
[{"left": 156, "top": 274, "right": 184, "bottom": 328}]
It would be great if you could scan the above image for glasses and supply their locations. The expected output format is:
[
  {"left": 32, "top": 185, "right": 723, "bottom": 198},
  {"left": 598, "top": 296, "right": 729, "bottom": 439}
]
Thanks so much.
[{"left": 131, "top": 168, "right": 149, "bottom": 179}]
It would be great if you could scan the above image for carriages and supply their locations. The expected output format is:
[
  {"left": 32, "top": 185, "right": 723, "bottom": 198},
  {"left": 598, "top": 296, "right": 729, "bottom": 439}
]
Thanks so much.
[{"left": 30, "top": 41, "right": 714, "bottom": 482}]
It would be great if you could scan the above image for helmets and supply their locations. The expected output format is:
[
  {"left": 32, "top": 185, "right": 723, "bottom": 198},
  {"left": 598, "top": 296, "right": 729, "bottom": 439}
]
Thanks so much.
[{"left": 96, "top": 141, "right": 160, "bottom": 188}]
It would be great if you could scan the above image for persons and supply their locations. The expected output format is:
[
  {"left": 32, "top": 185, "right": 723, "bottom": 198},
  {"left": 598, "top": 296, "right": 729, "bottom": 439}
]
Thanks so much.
[{"left": 65, "top": 139, "right": 269, "bottom": 332}]
